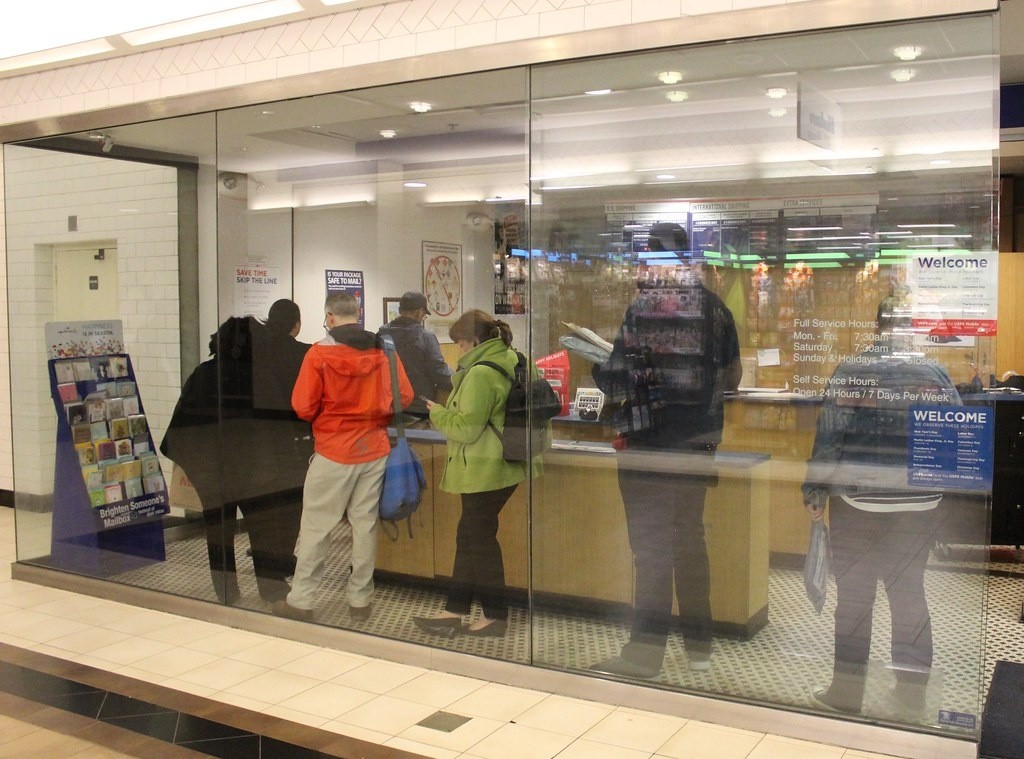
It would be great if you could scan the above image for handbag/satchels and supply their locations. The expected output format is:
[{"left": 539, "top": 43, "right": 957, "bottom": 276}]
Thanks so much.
[
  {"left": 803, "top": 491, "right": 828, "bottom": 616},
  {"left": 379, "top": 437, "right": 428, "bottom": 521}
]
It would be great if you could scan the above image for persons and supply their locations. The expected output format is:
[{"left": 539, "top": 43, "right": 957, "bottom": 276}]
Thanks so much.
[
  {"left": 375, "top": 290, "right": 455, "bottom": 419},
  {"left": 411, "top": 310, "right": 551, "bottom": 637},
  {"left": 590, "top": 220, "right": 744, "bottom": 679},
  {"left": 801, "top": 291, "right": 965, "bottom": 718},
  {"left": 269, "top": 292, "right": 416, "bottom": 619},
  {"left": 163, "top": 298, "right": 319, "bottom": 609}
]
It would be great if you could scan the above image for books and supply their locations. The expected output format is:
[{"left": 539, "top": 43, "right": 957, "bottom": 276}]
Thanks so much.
[{"left": 52, "top": 356, "right": 163, "bottom": 509}]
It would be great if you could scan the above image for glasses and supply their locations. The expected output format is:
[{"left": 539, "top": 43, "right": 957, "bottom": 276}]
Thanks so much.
[{"left": 323, "top": 312, "right": 336, "bottom": 333}]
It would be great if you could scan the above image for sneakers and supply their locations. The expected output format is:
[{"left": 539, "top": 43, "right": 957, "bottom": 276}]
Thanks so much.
[
  {"left": 810, "top": 684, "right": 862, "bottom": 715},
  {"left": 886, "top": 685, "right": 928, "bottom": 717}
]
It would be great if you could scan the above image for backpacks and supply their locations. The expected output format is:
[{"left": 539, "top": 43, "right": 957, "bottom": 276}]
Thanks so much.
[{"left": 472, "top": 352, "right": 562, "bottom": 462}]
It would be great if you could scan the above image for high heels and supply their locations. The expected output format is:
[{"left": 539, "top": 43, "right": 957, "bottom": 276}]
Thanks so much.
[
  {"left": 413, "top": 617, "right": 462, "bottom": 640},
  {"left": 461, "top": 618, "right": 508, "bottom": 638}
]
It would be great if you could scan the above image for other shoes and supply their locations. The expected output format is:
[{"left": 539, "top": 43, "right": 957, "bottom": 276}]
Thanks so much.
[
  {"left": 688, "top": 648, "right": 715, "bottom": 671},
  {"left": 588, "top": 654, "right": 661, "bottom": 682},
  {"left": 349, "top": 603, "right": 371, "bottom": 622},
  {"left": 263, "top": 601, "right": 314, "bottom": 622}
]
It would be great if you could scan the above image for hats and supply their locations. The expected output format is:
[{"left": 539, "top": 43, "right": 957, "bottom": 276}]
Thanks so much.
[
  {"left": 399, "top": 291, "right": 432, "bottom": 316},
  {"left": 647, "top": 223, "right": 689, "bottom": 251}
]
